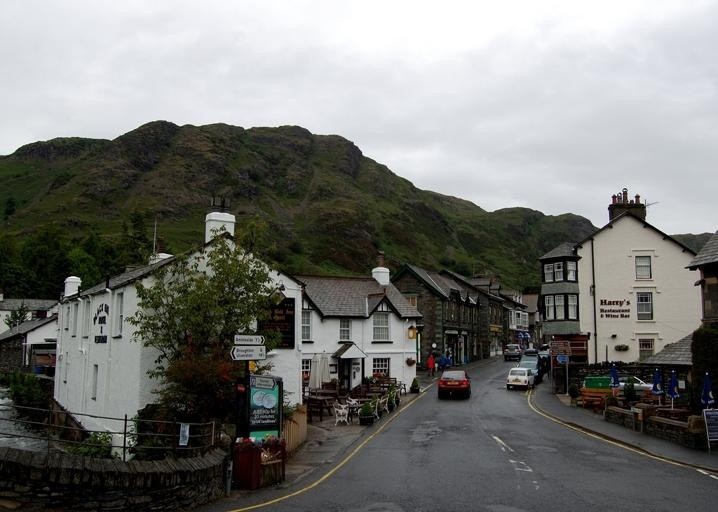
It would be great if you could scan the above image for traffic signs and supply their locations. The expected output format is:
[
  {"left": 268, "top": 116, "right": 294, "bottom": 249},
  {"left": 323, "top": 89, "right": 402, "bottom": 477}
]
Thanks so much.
[{"left": 230, "top": 334, "right": 267, "bottom": 360}]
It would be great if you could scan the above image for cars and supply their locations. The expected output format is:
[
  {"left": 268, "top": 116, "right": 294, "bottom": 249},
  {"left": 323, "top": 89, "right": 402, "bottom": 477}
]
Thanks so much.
[
  {"left": 618, "top": 376, "right": 654, "bottom": 394},
  {"left": 438, "top": 370, "right": 471, "bottom": 398},
  {"left": 504, "top": 344, "right": 522, "bottom": 360},
  {"left": 506, "top": 368, "right": 535, "bottom": 390}
]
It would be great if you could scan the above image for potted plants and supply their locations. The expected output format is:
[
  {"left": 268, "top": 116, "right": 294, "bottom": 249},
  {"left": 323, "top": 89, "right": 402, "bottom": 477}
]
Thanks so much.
[{"left": 410, "top": 377, "right": 420, "bottom": 393}]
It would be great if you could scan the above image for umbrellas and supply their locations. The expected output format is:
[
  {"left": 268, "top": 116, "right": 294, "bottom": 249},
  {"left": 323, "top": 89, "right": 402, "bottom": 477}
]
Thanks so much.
[
  {"left": 319, "top": 350, "right": 332, "bottom": 392},
  {"left": 699, "top": 372, "right": 716, "bottom": 409},
  {"left": 651, "top": 368, "right": 665, "bottom": 407},
  {"left": 608, "top": 363, "right": 621, "bottom": 397},
  {"left": 666, "top": 369, "right": 680, "bottom": 409},
  {"left": 307, "top": 353, "right": 322, "bottom": 400}
]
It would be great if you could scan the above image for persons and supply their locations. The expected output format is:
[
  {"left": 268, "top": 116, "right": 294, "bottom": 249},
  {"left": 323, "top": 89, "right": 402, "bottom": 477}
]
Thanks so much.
[{"left": 424, "top": 349, "right": 452, "bottom": 377}]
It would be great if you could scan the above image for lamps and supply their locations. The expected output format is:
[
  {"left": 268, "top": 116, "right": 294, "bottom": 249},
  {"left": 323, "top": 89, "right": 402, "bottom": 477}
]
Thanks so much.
[{"left": 408, "top": 326, "right": 417, "bottom": 340}]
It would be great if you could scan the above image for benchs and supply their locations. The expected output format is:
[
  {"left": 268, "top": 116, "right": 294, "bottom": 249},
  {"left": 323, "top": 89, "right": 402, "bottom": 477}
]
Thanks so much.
[
  {"left": 604, "top": 395, "right": 690, "bottom": 437},
  {"left": 306, "top": 378, "right": 406, "bottom": 426}
]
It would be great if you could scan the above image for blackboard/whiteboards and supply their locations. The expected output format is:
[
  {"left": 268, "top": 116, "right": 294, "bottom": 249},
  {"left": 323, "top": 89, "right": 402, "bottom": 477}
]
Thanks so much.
[{"left": 703, "top": 409, "right": 718, "bottom": 441}]
